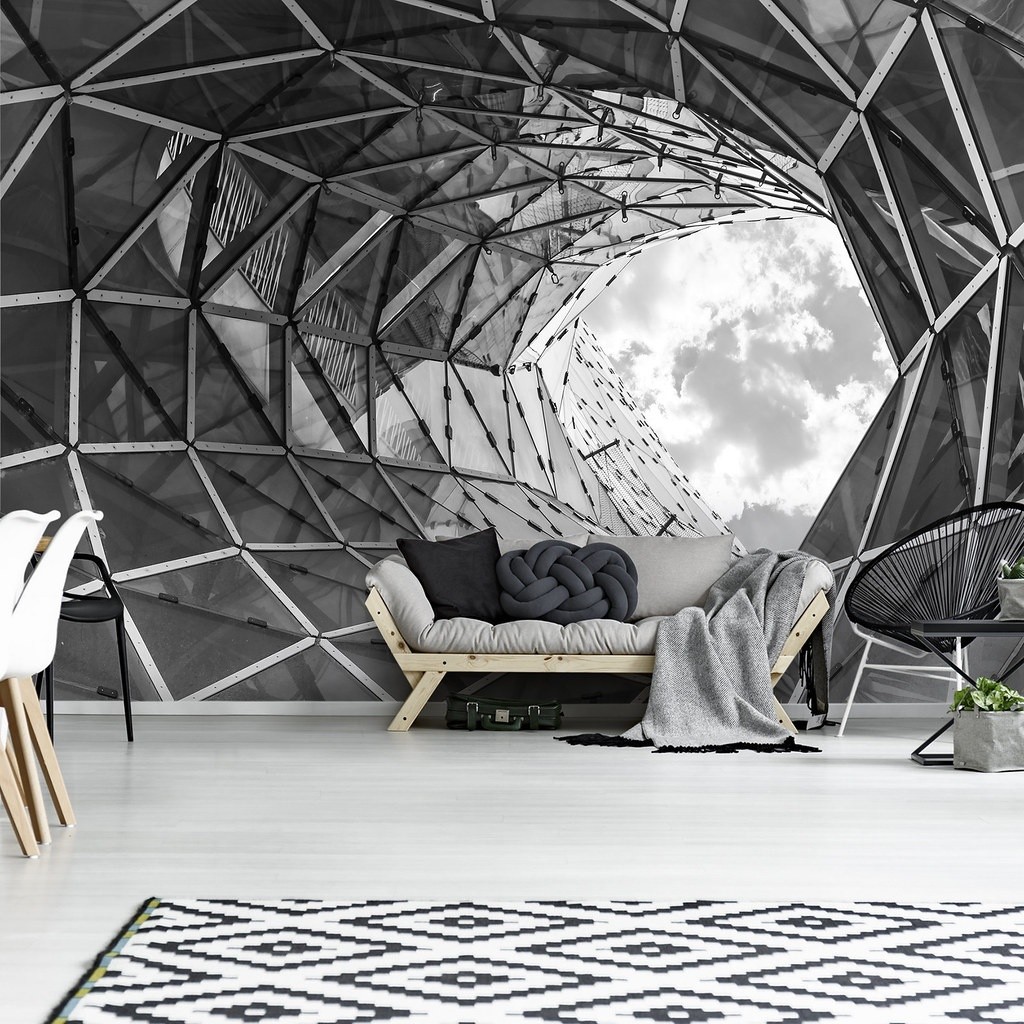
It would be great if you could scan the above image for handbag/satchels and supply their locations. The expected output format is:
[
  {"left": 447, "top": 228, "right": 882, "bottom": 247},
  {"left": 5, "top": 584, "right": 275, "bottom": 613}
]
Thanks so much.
[{"left": 444, "top": 693, "right": 561, "bottom": 731}]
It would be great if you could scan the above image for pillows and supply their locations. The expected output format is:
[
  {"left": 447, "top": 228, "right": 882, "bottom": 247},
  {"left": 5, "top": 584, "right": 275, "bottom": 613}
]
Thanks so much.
[
  {"left": 395, "top": 527, "right": 502, "bottom": 626},
  {"left": 435, "top": 535, "right": 589, "bottom": 557},
  {"left": 589, "top": 535, "right": 734, "bottom": 621},
  {"left": 498, "top": 540, "right": 638, "bottom": 625}
]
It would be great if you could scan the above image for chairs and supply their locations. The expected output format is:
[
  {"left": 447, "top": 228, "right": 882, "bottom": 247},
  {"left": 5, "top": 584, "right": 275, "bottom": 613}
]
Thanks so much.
[
  {"left": 845, "top": 502, "right": 1024, "bottom": 765},
  {"left": 0, "top": 508, "right": 103, "bottom": 857},
  {"left": 31, "top": 554, "right": 133, "bottom": 743}
]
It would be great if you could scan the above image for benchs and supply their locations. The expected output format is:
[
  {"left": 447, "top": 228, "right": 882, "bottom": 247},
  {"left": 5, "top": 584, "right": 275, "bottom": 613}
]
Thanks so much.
[{"left": 365, "top": 535, "right": 831, "bottom": 732}]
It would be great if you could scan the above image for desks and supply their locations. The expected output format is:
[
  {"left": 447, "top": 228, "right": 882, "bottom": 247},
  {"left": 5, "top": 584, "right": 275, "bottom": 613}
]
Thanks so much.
[{"left": 911, "top": 620, "right": 1024, "bottom": 765}]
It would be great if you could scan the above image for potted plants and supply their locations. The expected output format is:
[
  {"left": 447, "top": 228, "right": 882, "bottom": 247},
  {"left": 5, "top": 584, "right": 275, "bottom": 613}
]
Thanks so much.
[
  {"left": 946, "top": 676, "right": 1024, "bottom": 772},
  {"left": 993, "top": 557, "right": 1024, "bottom": 621}
]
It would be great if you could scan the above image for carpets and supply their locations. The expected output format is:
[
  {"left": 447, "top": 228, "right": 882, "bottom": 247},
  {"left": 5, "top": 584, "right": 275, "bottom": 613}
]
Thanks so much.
[{"left": 48, "top": 898, "right": 1024, "bottom": 1024}]
